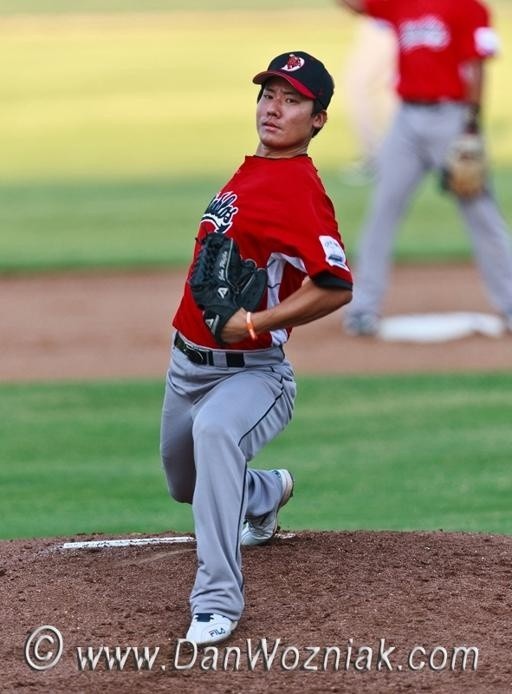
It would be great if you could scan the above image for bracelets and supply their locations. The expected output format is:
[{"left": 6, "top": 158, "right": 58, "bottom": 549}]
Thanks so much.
[{"left": 245, "top": 310, "right": 262, "bottom": 341}]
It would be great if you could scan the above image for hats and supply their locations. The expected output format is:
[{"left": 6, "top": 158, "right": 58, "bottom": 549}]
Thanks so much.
[{"left": 253, "top": 51, "right": 334, "bottom": 108}]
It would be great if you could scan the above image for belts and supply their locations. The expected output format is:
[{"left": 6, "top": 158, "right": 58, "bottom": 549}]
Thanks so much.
[{"left": 173, "top": 333, "right": 245, "bottom": 369}]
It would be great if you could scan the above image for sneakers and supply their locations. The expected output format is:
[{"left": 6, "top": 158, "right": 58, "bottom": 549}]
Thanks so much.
[
  {"left": 239, "top": 469, "right": 293, "bottom": 545},
  {"left": 186, "top": 612, "right": 239, "bottom": 647}
]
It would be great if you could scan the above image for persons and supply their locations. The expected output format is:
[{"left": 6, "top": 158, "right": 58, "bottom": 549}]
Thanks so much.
[
  {"left": 154, "top": 46, "right": 355, "bottom": 646},
  {"left": 338, "top": 1, "right": 512, "bottom": 343}
]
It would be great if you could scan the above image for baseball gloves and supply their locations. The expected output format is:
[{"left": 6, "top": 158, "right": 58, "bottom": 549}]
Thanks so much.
[
  {"left": 440, "top": 122, "right": 487, "bottom": 199},
  {"left": 190, "top": 233, "right": 267, "bottom": 348}
]
